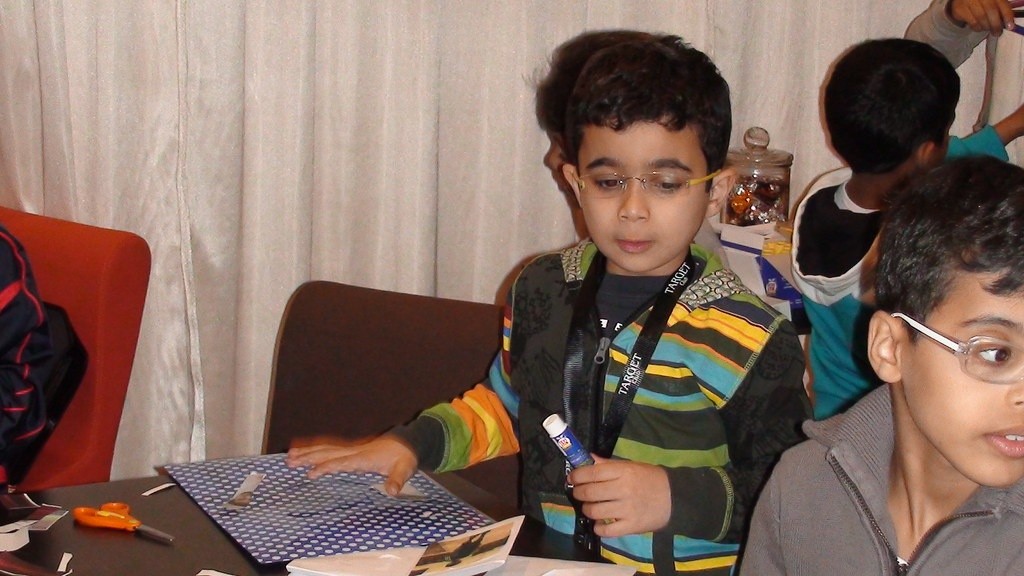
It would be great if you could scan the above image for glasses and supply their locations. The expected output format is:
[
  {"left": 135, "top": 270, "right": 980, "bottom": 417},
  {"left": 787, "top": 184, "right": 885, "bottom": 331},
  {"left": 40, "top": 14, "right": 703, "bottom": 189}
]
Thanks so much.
[
  {"left": 570, "top": 166, "right": 722, "bottom": 200},
  {"left": 890, "top": 312, "right": 1024, "bottom": 386}
]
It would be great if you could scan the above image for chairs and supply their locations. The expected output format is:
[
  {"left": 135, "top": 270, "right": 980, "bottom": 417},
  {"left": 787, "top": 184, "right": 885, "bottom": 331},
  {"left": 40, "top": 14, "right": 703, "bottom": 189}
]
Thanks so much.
[
  {"left": 0, "top": 206, "right": 153, "bottom": 493},
  {"left": 261, "top": 279, "right": 522, "bottom": 508}
]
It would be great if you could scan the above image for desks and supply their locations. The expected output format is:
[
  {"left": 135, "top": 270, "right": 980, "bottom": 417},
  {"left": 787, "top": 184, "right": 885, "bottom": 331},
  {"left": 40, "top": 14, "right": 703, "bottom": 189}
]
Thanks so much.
[{"left": 0, "top": 471, "right": 640, "bottom": 576}]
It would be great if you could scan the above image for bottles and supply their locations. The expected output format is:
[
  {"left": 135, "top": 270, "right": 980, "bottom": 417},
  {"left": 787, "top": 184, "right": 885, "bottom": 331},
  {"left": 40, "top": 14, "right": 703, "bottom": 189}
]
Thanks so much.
[{"left": 719, "top": 127, "right": 795, "bottom": 227}]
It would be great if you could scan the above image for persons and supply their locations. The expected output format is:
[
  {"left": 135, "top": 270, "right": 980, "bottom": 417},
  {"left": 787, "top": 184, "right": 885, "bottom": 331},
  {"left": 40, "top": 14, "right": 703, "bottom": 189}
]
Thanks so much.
[
  {"left": 740, "top": 155, "right": 1024, "bottom": 576},
  {"left": 790, "top": 0, "right": 1024, "bottom": 421},
  {"left": 284, "top": 31, "right": 814, "bottom": 576}
]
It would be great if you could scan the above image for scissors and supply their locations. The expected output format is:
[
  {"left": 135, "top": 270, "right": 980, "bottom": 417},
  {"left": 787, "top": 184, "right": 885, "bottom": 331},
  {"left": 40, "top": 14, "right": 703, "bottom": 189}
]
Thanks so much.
[{"left": 72, "top": 501, "right": 177, "bottom": 545}]
